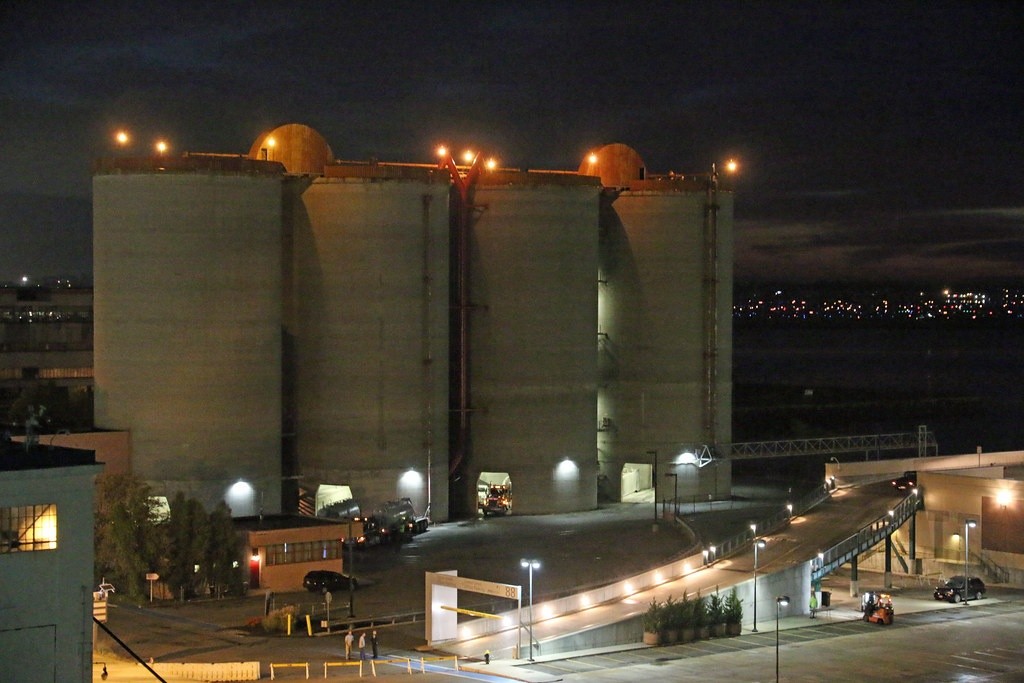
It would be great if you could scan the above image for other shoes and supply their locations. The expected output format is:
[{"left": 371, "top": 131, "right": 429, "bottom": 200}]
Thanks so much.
[{"left": 809, "top": 617, "right": 815, "bottom": 619}]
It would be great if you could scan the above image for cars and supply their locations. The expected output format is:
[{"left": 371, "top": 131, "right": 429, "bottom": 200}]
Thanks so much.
[{"left": 892, "top": 471, "right": 918, "bottom": 492}]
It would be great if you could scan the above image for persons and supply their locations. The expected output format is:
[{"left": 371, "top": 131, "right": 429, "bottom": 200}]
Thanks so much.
[
  {"left": 810, "top": 594, "right": 817, "bottom": 619},
  {"left": 345, "top": 630, "right": 354, "bottom": 659},
  {"left": 371, "top": 630, "right": 378, "bottom": 658},
  {"left": 359, "top": 633, "right": 366, "bottom": 659}
]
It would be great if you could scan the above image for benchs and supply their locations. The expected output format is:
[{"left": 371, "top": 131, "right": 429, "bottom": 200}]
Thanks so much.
[{"left": 810, "top": 607, "right": 833, "bottom": 620}]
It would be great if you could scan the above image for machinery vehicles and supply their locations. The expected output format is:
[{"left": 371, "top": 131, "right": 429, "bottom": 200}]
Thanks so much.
[{"left": 855, "top": 591, "right": 894, "bottom": 625}]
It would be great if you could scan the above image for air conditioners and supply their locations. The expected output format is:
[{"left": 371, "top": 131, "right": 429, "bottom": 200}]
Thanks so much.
[{"left": 662, "top": 594, "right": 682, "bottom": 643}]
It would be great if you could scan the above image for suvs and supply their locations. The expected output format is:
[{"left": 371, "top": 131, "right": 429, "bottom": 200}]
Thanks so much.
[
  {"left": 934, "top": 575, "right": 986, "bottom": 604},
  {"left": 303, "top": 570, "right": 359, "bottom": 593}
]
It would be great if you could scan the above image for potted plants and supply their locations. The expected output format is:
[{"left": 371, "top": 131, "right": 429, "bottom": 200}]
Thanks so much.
[
  {"left": 680, "top": 590, "right": 696, "bottom": 640},
  {"left": 707, "top": 585, "right": 727, "bottom": 637},
  {"left": 642, "top": 596, "right": 663, "bottom": 644},
  {"left": 695, "top": 587, "right": 714, "bottom": 639},
  {"left": 724, "top": 586, "right": 743, "bottom": 635}
]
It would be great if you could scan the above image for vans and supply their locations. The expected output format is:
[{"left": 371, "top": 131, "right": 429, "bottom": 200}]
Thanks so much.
[{"left": 478, "top": 485, "right": 489, "bottom": 504}]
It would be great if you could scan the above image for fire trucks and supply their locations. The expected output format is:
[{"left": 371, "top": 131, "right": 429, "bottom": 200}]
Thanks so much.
[{"left": 483, "top": 484, "right": 511, "bottom": 516}]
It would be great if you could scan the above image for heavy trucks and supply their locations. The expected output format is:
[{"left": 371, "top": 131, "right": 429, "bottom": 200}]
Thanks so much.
[{"left": 342, "top": 498, "right": 428, "bottom": 549}]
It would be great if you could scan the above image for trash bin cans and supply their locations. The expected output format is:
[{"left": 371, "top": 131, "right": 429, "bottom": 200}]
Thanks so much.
[{"left": 821, "top": 591, "right": 833, "bottom": 607}]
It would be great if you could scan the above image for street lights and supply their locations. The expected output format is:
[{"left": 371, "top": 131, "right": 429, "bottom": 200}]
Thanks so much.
[
  {"left": 665, "top": 472, "right": 678, "bottom": 518},
  {"left": 776, "top": 596, "right": 791, "bottom": 683},
  {"left": 520, "top": 559, "right": 540, "bottom": 662},
  {"left": 754, "top": 539, "right": 766, "bottom": 632},
  {"left": 648, "top": 450, "right": 658, "bottom": 522},
  {"left": 966, "top": 519, "right": 976, "bottom": 606}
]
